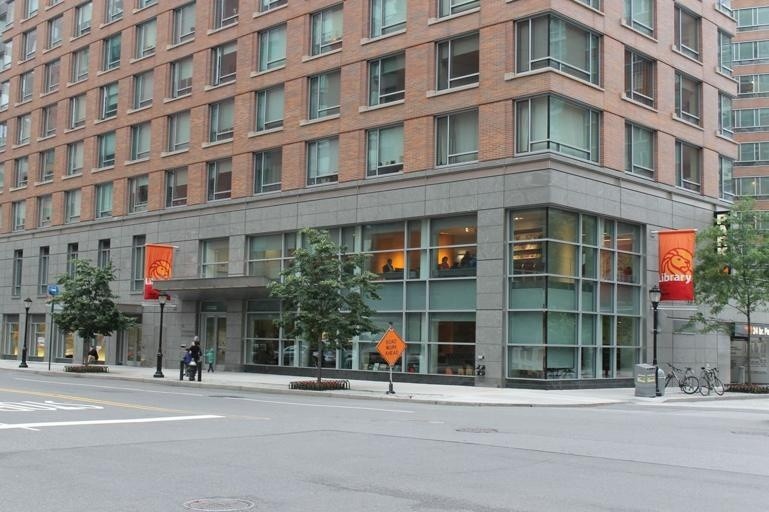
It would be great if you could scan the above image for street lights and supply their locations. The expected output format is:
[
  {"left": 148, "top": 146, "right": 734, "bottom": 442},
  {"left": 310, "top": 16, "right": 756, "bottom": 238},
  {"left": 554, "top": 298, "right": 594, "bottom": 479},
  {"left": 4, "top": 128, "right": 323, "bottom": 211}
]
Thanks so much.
[
  {"left": 649, "top": 285, "right": 662, "bottom": 396},
  {"left": 154, "top": 289, "right": 170, "bottom": 378},
  {"left": 18, "top": 296, "right": 34, "bottom": 367}
]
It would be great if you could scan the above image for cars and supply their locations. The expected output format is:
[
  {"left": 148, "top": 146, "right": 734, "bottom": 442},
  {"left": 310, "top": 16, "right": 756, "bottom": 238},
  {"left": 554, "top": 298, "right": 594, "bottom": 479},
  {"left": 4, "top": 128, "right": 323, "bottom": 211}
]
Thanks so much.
[
  {"left": 252, "top": 341, "right": 426, "bottom": 369},
  {"left": 36, "top": 332, "right": 142, "bottom": 364}
]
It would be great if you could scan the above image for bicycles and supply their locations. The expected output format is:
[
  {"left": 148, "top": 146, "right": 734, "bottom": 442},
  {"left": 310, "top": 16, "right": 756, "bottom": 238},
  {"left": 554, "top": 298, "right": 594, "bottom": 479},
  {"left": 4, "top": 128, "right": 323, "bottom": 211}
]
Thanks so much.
[
  {"left": 698, "top": 367, "right": 725, "bottom": 396},
  {"left": 664, "top": 362, "right": 700, "bottom": 394}
]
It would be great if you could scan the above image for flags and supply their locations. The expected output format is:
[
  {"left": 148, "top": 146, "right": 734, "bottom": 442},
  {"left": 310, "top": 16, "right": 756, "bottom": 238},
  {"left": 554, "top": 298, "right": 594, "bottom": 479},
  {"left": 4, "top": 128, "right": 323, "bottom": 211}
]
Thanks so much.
[
  {"left": 144, "top": 243, "right": 174, "bottom": 301},
  {"left": 657, "top": 229, "right": 695, "bottom": 302}
]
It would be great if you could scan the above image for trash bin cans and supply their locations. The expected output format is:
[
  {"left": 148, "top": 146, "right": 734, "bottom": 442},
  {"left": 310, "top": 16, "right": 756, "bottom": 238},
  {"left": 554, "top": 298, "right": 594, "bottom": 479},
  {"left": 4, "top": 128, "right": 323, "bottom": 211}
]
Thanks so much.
[{"left": 634, "top": 363, "right": 666, "bottom": 398}]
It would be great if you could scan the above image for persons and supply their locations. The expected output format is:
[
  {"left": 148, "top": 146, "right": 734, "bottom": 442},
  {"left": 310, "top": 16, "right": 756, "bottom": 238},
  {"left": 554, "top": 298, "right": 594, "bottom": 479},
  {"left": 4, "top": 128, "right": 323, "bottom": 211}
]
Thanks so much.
[
  {"left": 182, "top": 348, "right": 190, "bottom": 374},
  {"left": 201, "top": 348, "right": 215, "bottom": 372},
  {"left": 460, "top": 251, "right": 473, "bottom": 266},
  {"left": 185, "top": 336, "right": 203, "bottom": 381},
  {"left": 382, "top": 258, "right": 395, "bottom": 273},
  {"left": 437, "top": 256, "right": 449, "bottom": 268},
  {"left": 88, "top": 345, "right": 99, "bottom": 362}
]
